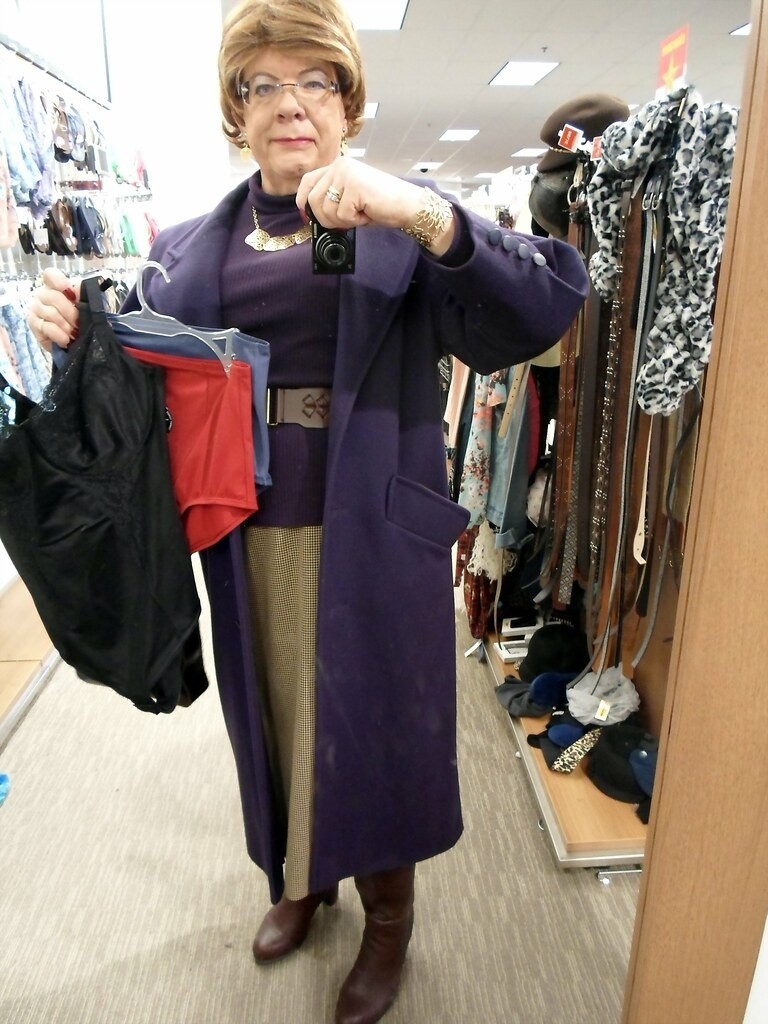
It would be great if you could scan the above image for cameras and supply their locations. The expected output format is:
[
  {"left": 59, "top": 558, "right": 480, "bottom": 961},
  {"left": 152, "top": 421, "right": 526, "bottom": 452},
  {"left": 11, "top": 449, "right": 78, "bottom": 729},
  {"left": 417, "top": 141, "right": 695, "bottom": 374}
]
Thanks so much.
[{"left": 306, "top": 202, "right": 355, "bottom": 276}]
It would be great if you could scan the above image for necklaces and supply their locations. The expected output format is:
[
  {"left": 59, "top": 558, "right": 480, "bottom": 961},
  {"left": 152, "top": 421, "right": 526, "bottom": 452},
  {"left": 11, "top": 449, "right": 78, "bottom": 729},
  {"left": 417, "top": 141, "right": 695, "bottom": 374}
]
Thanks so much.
[{"left": 245, "top": 205, "right": 316, "bottom": 251}]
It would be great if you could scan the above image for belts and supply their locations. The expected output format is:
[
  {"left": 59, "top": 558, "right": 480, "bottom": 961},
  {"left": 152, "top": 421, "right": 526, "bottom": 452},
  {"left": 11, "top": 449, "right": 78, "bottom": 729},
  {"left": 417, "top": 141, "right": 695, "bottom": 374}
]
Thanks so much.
[
  {"left": 265, "top": 387, "right": 332, "bottom": 428},
  {"left": 528, "top": 117, "right": 702, "bottom": 696}
]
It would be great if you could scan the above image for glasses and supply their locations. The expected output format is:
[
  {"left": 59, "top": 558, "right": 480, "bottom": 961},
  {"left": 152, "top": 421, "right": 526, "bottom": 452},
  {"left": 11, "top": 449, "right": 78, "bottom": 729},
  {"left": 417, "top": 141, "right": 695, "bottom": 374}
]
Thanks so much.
[{"left": 237, "top": 72, "right": 341, "bottom": 105}]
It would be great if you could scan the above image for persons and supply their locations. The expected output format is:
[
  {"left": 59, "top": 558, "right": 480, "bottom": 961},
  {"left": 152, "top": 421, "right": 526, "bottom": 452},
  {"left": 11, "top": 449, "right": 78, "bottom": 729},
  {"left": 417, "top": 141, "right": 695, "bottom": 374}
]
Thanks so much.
[{"left": 27, "top": 0, "right": 590, "bottom": 1024}]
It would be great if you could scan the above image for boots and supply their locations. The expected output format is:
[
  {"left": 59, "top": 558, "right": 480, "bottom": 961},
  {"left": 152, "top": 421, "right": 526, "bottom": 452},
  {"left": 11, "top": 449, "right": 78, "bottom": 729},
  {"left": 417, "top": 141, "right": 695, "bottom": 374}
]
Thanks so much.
[
  {"left": 334, "top": 862, "right": 415, "bottom": 1024},
  {"left": 252, "top": 878, "right": 339, "bottom": 963}
]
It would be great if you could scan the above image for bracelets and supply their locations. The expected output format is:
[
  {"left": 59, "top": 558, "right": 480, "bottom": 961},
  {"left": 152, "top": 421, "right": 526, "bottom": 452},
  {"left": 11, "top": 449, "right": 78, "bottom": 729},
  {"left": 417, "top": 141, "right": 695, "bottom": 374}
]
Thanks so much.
[{"left": 399, "top": 185, "right": 453, "bottom": 250}]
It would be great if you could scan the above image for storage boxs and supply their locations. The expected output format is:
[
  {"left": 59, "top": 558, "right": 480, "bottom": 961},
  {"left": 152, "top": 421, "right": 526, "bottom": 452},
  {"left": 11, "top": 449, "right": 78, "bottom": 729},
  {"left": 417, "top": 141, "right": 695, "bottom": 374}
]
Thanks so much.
[{"left": 494, "top": 617, "right": 544, "bottom": 664}]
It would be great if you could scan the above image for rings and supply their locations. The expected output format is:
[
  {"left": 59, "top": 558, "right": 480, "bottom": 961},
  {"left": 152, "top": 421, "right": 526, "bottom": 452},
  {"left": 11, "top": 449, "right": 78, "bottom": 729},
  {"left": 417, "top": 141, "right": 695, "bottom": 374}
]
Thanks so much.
[
  {"left": 326, "top": 186, "right": 342, "bottom": 203},
  {"left": 37, "top": 319, "right": 45, "bottom": 336}
]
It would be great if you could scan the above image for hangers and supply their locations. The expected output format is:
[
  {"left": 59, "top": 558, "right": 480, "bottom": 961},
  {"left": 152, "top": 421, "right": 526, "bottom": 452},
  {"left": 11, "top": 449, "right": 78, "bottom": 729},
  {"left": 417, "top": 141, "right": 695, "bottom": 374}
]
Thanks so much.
[{"left": 0, "top": 46, "right": 240, "bottom": 381}]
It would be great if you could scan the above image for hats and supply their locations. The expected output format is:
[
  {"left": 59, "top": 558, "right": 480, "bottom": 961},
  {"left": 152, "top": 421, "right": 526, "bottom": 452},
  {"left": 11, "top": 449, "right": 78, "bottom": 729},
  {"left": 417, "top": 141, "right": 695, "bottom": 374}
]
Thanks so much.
[
  {"left": 495, "top": 624, "right": 659, "bottom": 823},
  {"left": 529, "top": 93, "right": 630, "bottom": 240}
]
